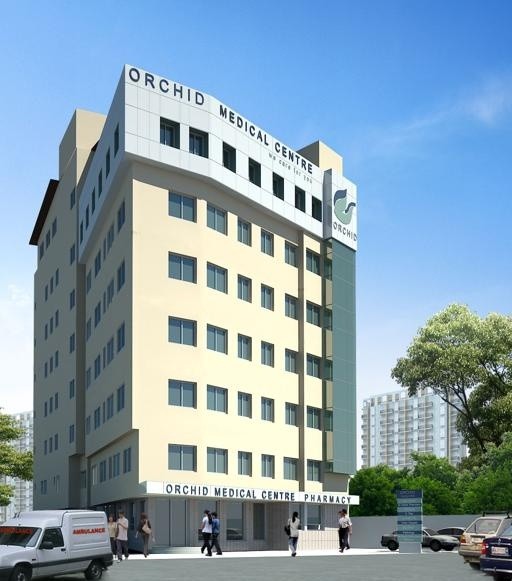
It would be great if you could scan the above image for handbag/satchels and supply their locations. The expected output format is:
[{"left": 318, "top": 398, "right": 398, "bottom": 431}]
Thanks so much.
[
  {"left": 142, "top": 520, "right": 152, "bottom": 535},
  {"left": 284, "top": 525, "right": 291, "bottom": 536}
]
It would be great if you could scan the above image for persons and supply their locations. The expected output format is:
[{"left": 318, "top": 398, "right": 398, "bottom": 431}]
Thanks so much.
[
  {"left": 135, "top": 513, "right": 153, "bottom": 558},
  {"left": 201, "top": 509, "right": 212, "bottom": 556},
  {"left": 338, "top": 509, "right": 352, "bottom": 553},
  {"left": 288, "top": 511, "right": 300, "bottom": 557},
  {"left": 210, "top": 512, "right": 222, "bottom": 555},
  {"left": 108, "top": 509, "right": 129, "bottom": 563}
]
